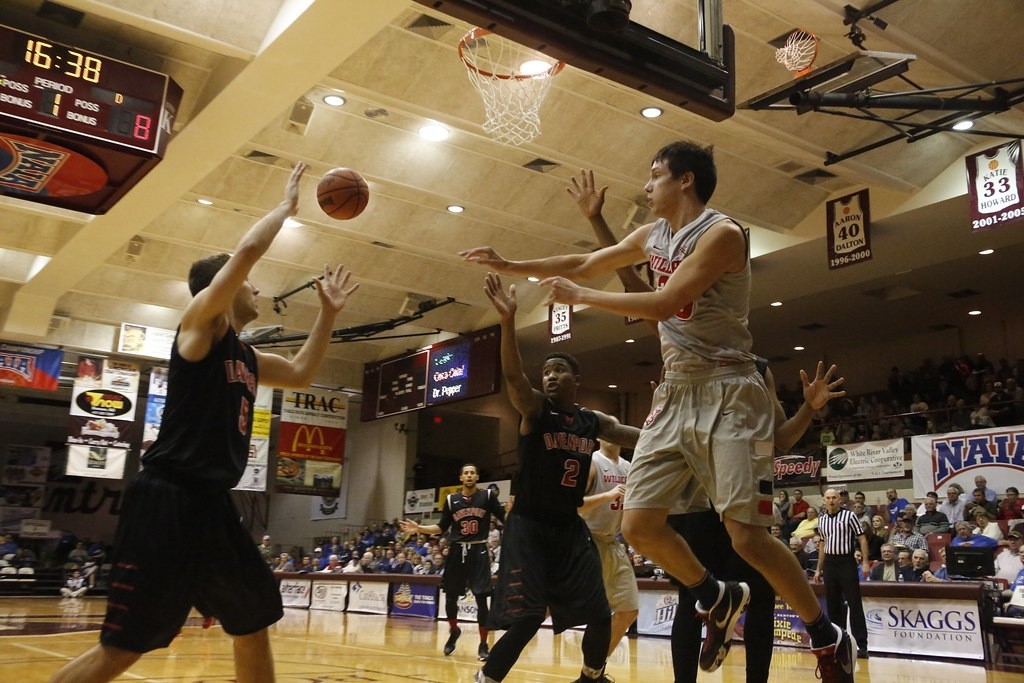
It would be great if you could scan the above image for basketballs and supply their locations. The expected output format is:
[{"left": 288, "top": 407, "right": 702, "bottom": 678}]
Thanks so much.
[{"left": 316, "top": 167, "right": 371, "bottom": 222}]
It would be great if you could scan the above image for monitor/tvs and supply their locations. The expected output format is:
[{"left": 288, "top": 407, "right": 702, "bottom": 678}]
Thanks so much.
[{"left": 944, "top": 546, "right": 996, "bottom": 581}]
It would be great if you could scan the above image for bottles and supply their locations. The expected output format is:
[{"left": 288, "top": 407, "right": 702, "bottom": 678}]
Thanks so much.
[{"left": 898, "top": 573, "right": 904, "bottom": 582}]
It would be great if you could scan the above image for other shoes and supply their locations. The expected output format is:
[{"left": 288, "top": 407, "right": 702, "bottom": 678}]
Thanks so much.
[
  {"left": 582, "top": 662, "right": 616, "bottom": 683},
  {"left": 474, "top": 667, "right": 501, "bottom": 683},
  {"left": 856, "top": 649, "right": 869, "bottom": 658}
]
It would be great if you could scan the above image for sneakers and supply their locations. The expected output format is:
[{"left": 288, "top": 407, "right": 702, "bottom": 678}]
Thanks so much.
[
  {"left": 809, "top": 622, "right": 856, "bottom": 683},
  {"left": 444, "top": 627, "right": 461, "bottom": 655},
  {"left": 478, "top": 643, "right": 489, "bottom": 660},
  {"left": 694, "top": 578, "right": 750, "bottom": 672}
]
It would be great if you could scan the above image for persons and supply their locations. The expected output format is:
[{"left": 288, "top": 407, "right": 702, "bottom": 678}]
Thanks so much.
[
  {"left": 576, "top": 416, "right": 639, "bottom": 659},
  {"left": 471, "top": 272, "right": 641, "bottom": 683},
  {"left": 50, "top": 162, "right": 361, "bottom": 683},
  {"left": 617, "top": 475, "right": 1023, "bottom": 613},
  {"left": 812, "top": 489, "right": 870, "bottom": 659},
  {"left": 0, "top": 530, "right": 106, "bottom": 598},
  {"left": 254, "top": 516, "right": 504, "bottom": 576},
  {"left": 458, "top": 141, "right": 857, "bottom": 683},
  {"left": 396, "top": 465, "right": 516, "bottom": 661}
]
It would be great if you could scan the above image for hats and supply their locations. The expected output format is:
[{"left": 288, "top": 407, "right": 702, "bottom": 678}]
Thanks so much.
[
  {"left": 901, "top": 515, "right": 913, "bottom": 521},
  {"left": 314, "top": 548, "right": 321, "bottom": 552},
  {"left": 263, "top": 535, "right": 270, "bottom": 540},
  {"left": 1008, "top": 531, "right": 1023, "bottom": 538}
]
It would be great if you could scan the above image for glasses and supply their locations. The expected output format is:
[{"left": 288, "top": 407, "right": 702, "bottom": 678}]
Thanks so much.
[{"left": 902, "top": 520, "right": 913, "bottom": 524}]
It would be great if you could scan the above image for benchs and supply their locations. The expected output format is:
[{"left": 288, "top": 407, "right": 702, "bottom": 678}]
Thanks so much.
[{"left": 0, "top": 539, "right": 109, "bottom": 596}]
